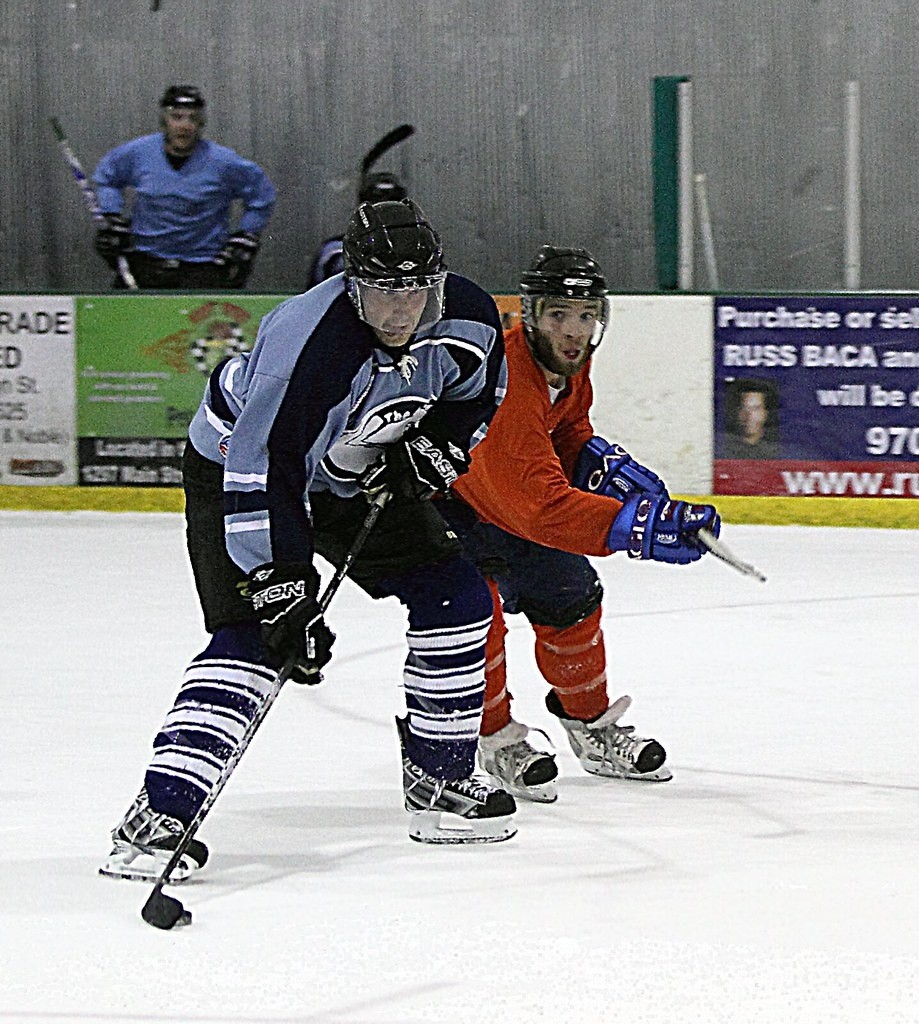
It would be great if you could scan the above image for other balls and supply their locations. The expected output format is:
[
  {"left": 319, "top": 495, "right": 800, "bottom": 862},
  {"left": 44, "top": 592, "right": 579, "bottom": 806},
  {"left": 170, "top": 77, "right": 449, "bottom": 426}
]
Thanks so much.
[{"left": 173, "top": 910, "right": 194, "bottom": 928}]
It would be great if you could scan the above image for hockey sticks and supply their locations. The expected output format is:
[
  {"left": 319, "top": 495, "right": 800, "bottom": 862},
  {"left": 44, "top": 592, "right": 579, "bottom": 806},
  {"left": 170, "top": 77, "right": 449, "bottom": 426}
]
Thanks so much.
[
  {"left": 694, "top": 523, "right": 769, "bottom": 586},
  {"left": 356, "top": 122, "right": 419, "bottom": 185},
  {"left": 138, "top": 483, "right": 395, "bottom": 932},
  {"left": 45, "top": 114, "right": 143, "bottom": 293}
]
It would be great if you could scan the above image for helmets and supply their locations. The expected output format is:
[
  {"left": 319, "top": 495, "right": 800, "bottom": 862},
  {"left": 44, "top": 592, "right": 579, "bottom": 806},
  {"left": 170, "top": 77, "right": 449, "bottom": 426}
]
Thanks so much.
[
  {"left": 343, "top": 197, "right": 447, "bottom": 291},
  {"left": 161, "top": 85, "right": 206, "bottom": 110},
  {"left": 359, "top": 171, "right": 407, "bottom": 204},
  {"left": 520, "top": 244, "right": 609, "bottom": 300}
]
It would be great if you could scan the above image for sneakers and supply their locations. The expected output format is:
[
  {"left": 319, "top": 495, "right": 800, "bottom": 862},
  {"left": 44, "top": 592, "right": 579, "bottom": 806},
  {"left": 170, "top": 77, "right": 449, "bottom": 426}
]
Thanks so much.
[
  {"left": 478, "top": 718, "right": 560, "bottom": 803},
  {"left": 546, "top": 687, "right": 673, "bottom": 781},
  {"left": 394, "top": 712, "right": 517, "bottom": 844},
  {"left": 97, "top": 786, "right": 209, "bottom": 883}
]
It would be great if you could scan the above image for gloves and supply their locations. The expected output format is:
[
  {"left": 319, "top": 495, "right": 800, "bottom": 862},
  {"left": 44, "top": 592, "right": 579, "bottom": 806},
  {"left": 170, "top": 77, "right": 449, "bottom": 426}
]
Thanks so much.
[
  {"left": 572, "top": 436, "right": 669, "bottom": 500},
  {"left": 249, "top": 561, "right": 335, "bottom": 685},
  {"left": 96, "top": 213, "right": 136, "bottom": 272},
  {"left": 607, "top": 492, "right": 721, "bottom": 565},
  {"left": 359, "top": 428, "right": 471, "bottom": 504},
  {"left": 213, "top": 233, "right": 262, "bottom": 289}
]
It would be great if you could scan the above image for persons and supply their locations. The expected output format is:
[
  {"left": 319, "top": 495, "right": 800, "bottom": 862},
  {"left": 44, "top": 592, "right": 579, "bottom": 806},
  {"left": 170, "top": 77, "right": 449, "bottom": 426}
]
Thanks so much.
[
  {"left": 709, "top": 380, "right": 791, "bottom": 466},
  {"left": 92, "top": 85, "right": 274, "bottom": 293},
  {"left": 97, "top": 194, "right": 522, "bottom": 883},
  {"left": 434, "top": 244, "right": 725, "bottom": 802}
]
